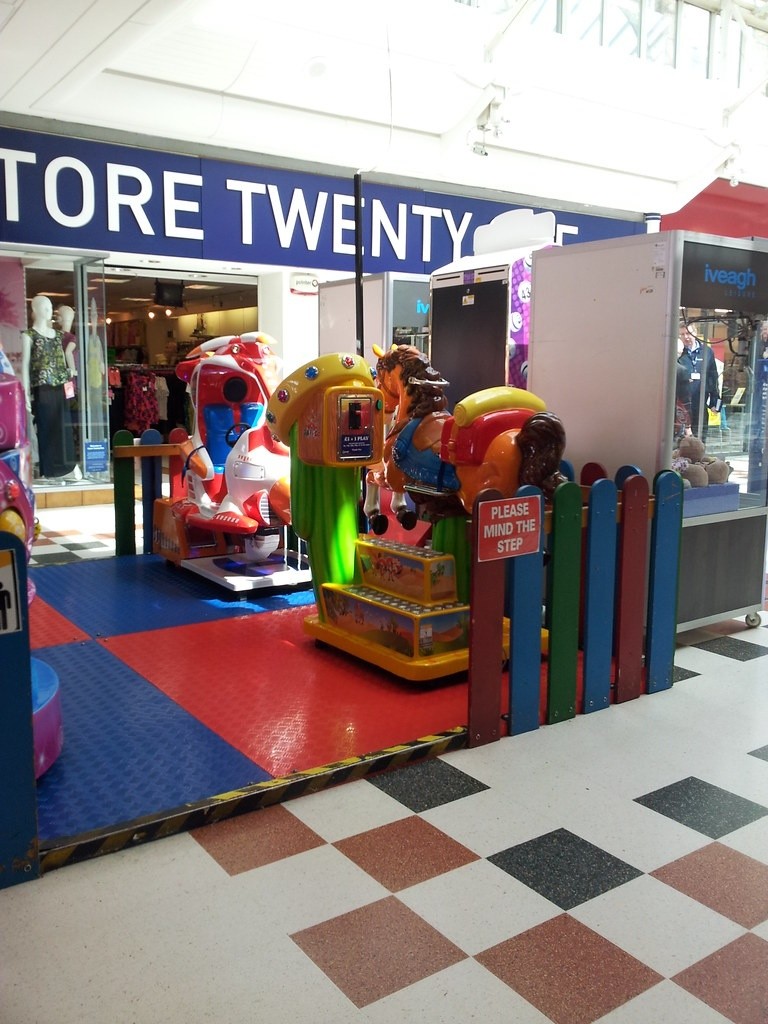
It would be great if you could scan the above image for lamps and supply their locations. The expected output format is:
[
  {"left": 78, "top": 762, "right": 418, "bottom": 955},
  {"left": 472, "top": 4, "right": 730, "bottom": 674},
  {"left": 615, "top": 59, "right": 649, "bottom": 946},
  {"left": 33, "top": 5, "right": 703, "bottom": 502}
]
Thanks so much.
[{"left": 154, "top": 281, "right": 185, "bottom": 307}]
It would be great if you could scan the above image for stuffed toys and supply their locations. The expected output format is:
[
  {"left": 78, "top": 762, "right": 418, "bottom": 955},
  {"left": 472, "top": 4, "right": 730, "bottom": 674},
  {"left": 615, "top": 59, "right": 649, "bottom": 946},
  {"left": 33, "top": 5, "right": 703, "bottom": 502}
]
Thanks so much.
[{"left": 669, "top": 435, "right": 734, "bottom": 489}]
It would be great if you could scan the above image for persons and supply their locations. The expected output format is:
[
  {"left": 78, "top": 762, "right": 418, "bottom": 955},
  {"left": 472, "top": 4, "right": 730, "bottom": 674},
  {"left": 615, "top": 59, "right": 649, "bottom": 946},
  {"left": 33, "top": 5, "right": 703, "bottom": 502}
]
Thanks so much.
[
  {"left": 678, "top": 320, "right": 719, "bottom": 447},
  {"left": 20, "top": 294, "right": 83, "bottom": 485},
  {"left": 52, "top": 305, "right": 79, "bottom": 461},
  {"left": 758, "top": 321, "right": 767, "bottom": 359},
  {"left": 675, "top": 339, "right": 694, "bottom": 440},
  {"left": 84, "top": 296, "right": 107, "bottom": 480}
]
